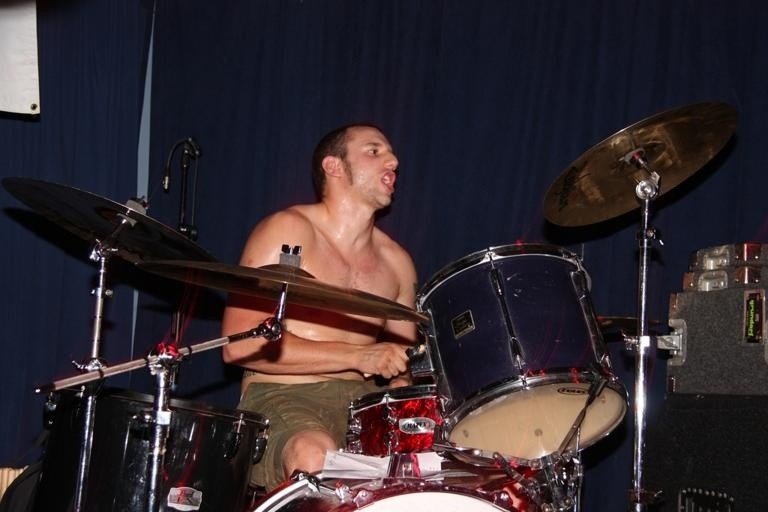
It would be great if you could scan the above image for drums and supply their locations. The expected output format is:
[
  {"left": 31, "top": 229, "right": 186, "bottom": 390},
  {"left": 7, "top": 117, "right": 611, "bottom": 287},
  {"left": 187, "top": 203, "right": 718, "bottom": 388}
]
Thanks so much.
[
  {"left": 348, "top": 385, "right": 551, "bottom": 512},
  {"left": 28, "top": 381, "right": 271, "bottom": 510},
  {"left": 416, "top": 242, "right": 629, "bottom": 470},
  {"left": 248, "top": 470, "right": 522, "bottom": 512}
]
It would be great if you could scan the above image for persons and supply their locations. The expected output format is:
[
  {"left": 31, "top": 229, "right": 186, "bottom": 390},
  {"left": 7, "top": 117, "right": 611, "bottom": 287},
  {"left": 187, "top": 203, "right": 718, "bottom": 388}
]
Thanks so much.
[{"left": 220, "top": 123, "right": 422, "bottom": 495}]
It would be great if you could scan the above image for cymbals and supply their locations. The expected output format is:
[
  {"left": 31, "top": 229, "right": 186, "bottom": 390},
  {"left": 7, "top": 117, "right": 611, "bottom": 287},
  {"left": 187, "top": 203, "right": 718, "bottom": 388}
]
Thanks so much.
[
  {"left": 542, "top": 103, "right": 739, "bottom": 227},
  {"left": 5, "top": 176, "right": 218, "bottom": 262},
  {"left": 116, "top": 263, "right": 428, "bottom": 322},
  {"left": 598, "top": 315, "right": 661, "bottom": 342}
]
